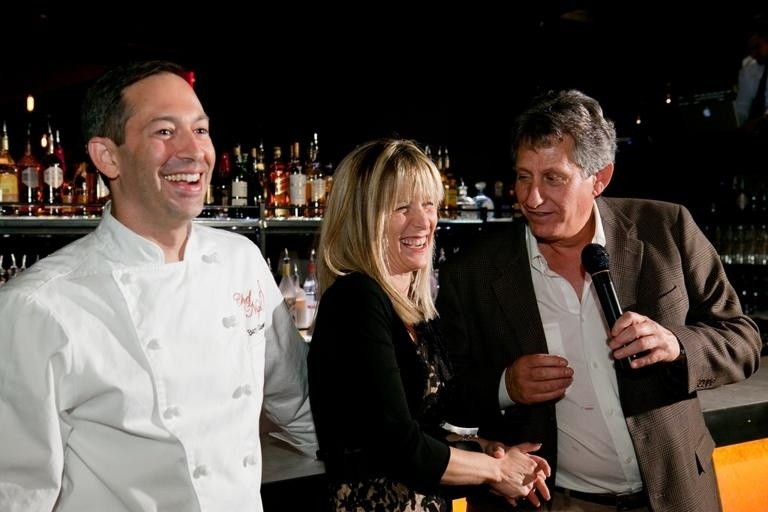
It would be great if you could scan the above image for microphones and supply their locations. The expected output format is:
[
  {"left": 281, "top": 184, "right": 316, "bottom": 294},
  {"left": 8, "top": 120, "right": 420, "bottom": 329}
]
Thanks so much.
[{"left": 581, "top": 243, "right": 637, "bottom": 370}]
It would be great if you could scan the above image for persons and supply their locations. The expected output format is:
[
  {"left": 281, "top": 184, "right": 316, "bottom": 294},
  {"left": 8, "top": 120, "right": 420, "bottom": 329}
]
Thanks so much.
[
  {"left": 426, "top": 92, "right": 764, "bottom": 511},
  {"left": 0, "top": 60, "right": 326, "bottom": 511},
  {"left": 308, "top": 140, "right": 550, "bottom": 512}
]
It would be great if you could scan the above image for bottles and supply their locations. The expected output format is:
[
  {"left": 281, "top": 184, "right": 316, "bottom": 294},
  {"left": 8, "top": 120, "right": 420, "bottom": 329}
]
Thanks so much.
[
  {"left": 1, "top": 96, "right": 455, "bottom": 220},
  {"left": 272, "top": 245, "right": 320, "bottom": 335},
  {"left": 697, "top": 170, "right": 768, "bottom": 268}
]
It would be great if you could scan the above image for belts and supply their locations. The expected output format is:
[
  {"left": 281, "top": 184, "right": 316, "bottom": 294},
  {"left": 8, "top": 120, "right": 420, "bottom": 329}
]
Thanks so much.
[{"left": 552, "top": 484, "right": 651, "bottom": 509}]
